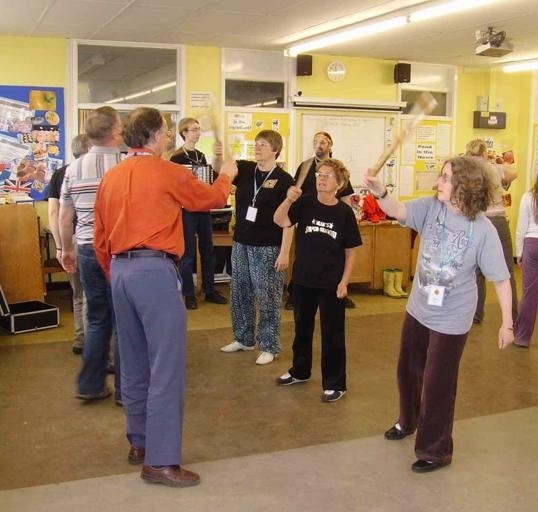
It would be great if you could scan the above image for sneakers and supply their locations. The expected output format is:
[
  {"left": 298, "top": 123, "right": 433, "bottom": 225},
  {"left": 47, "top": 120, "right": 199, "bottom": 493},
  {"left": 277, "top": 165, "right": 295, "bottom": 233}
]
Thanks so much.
[
  {"left": 321, "top": 389, "right": 346, "bottom": 403},
  {"left": 412, "top": 460, "right": 453, "bottom": 473},
  {"left": 205, "top": 291, "right": 228, "bottom": 304},
  {"left": 275, "top": 373, "right": 311, "bottom": 386},
  {"left": 184, "top": 295, "right": 197, "bottom": 309},
  {"left": 384, "top": 425, "right": 407, "bottom": 439},
  {"left": 104, "top": 356, "right": 117, "bottom": 373},
  {"left": 74, "top": 387, "right": 111, "bottom": 400},
  {"left": 255, "top": 350, "right": 278, "bottom": 364},
  {"left": 220, "top": 340, "right": 254, "bottom": 353}
]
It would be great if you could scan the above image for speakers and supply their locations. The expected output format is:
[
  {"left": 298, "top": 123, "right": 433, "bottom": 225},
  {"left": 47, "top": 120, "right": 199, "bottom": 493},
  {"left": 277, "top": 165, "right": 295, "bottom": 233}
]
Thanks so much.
[
  {"left": 297, "top": 55, "right": 313, "bottom": 76},
  {"left": 394, "top": 63, "right": 412, "bottom": 84}
]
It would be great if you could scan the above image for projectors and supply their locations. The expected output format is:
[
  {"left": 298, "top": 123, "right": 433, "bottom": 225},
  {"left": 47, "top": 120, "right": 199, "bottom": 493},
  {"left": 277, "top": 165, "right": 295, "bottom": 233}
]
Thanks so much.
[{"left": 475, "top": 42, "right": 513, "bottom": 58}]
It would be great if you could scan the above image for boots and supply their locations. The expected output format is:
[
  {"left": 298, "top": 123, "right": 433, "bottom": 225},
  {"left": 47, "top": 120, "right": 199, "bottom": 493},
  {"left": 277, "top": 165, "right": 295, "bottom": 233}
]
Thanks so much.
[
  {"left": 383, "top": 269, "right": 401, "bottom": 298},
  {"left": 395, "top": 269, "right": 408, "bottom": 298}
]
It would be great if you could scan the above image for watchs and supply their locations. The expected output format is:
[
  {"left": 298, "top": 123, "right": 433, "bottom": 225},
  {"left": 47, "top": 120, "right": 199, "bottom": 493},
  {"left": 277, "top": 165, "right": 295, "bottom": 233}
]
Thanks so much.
[
  {"left": 55, "top": 247, "right": 62, "bottom": 250},
  {"left": 375, "top": 189, "right": 388, "bottom": 200}
]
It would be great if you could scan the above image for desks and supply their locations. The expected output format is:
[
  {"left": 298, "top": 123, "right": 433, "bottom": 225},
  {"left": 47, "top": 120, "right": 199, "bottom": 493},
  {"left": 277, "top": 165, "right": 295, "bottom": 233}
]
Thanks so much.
[{"left": 1, "top": 203, "right": 47, "bottom": 305}]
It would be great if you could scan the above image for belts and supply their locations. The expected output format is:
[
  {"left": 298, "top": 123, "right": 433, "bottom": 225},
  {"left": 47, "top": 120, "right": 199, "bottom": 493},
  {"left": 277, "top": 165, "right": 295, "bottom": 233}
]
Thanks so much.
[{"left": 109, "top": 249, "right": 173, "bottom": 259}]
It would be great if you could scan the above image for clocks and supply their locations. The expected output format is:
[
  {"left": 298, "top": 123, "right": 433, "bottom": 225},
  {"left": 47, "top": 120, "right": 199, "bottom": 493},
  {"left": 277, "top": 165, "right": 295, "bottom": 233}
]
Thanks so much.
[{"left": 327, "top": 60, "right": 346, "bottom": 82}]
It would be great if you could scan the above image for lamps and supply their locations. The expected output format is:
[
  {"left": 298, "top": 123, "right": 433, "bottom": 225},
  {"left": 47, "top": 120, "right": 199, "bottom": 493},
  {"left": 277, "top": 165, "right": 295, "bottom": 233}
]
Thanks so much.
[
  {"left": 279, "top": 2, "right": 488, "bottom": 58},
  {"left": 502, "top": 58, "right": 534, "bottom": 74}
]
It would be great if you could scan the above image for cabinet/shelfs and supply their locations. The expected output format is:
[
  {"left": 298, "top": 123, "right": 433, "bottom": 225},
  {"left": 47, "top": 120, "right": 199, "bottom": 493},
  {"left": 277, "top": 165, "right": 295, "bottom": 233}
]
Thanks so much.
[
  {"left": 194, "top": 234, "right": 234, "bottom": 297},
  {"left": 348, "top": 224, "right": 412, "bottom": 294}
]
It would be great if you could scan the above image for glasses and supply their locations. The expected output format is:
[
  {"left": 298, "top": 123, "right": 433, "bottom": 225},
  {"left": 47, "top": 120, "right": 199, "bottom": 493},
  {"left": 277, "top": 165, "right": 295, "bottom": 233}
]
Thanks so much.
[
  {"left": 438, "top": 174, "right": 459, "bottom": 182},
  {"left": 160, "top": 129, "right": 172, "bottom": 135},
  {"left": 185, "top": 128, "right": 201, "bottom": 133},
  {"left": 253, "top": 143, "right": 272, "bottom": 148},
  {"left": 315, "top": 173, "right": 338, "bottom": 179},
  {"left": 313, "top": 139, "right": 329, "bottom": 145}
]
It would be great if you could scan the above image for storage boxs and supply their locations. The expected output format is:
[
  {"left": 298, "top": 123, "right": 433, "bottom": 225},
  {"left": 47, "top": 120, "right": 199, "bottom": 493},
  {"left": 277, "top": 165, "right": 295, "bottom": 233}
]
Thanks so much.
[{"left": 0, "top": 282, "right": 59, "bottom": 336}]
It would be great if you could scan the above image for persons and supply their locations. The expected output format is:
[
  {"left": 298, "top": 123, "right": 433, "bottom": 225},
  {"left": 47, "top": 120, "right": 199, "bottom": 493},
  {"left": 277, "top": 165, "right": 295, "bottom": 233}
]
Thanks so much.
[
  {"left": 467, "top": 139, "right": 518, "bottom": 331},
  {"left": 362, "top": 157, "right": 516, "bottom": 472},
  {"left": 93, "top": 106, "right": 238, "bottom": 489},
  {"left": 273, "top": 159, "right": 363, "bottom": 402},
  {"left": 169, "top": 117, "right": 229, "bottom": 309},
  {"left": 286, "top": 132, "right": 356, "bottom": 309},
  {"left": 41, "top": 134, "right": 114, "bottom": 375},
  {"left": 515, "top": 175, "right": 538, "bottom": 348},
  {"left": 59, "top": 106, "right": 128, "bottom": 408},
  {"left": 211, "top": 130, "right": 296, "bottom": 365}
]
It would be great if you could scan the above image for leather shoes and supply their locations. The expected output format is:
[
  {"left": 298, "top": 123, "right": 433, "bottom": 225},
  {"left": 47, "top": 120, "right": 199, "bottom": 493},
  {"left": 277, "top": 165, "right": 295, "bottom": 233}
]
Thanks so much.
[
  {"left": 140, "top": 465, "right": 200, "bottom": 486},
  {"left": 129, "top": 446, "right": 146, "bottom": 465},
  {"left": 72, "top": 344, "right": 84, "bottom": 354}
]
